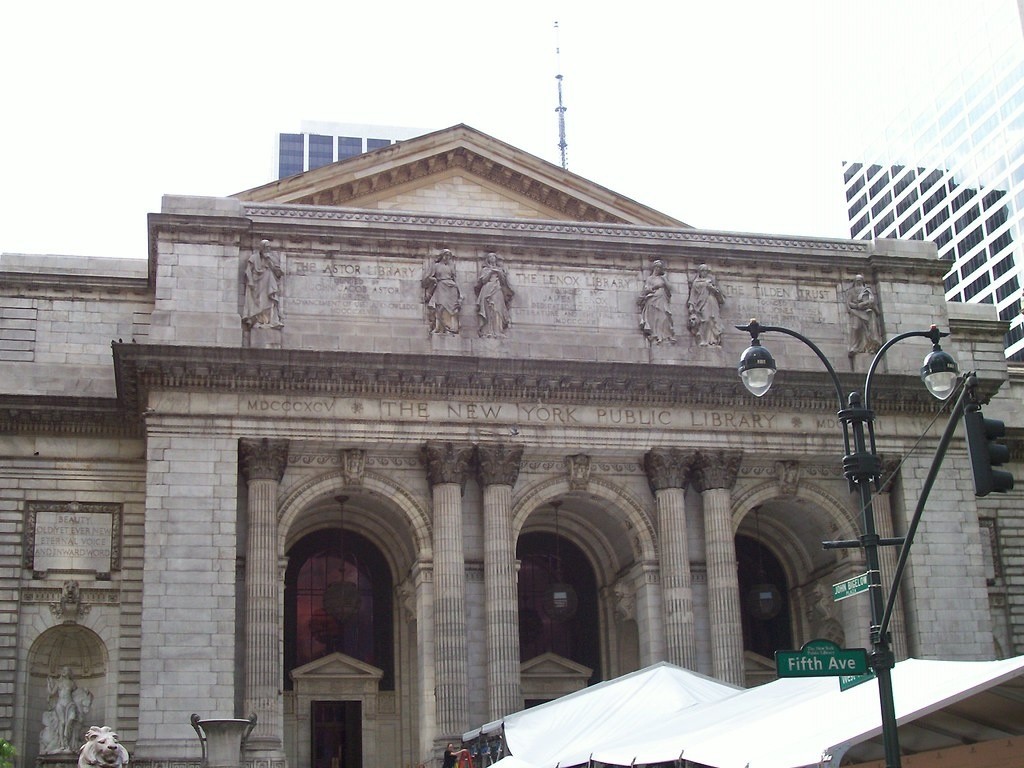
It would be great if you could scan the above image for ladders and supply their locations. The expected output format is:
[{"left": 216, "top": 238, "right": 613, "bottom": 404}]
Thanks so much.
[{"left": 458, "top": 751, "right": 473, "bottom": 768}]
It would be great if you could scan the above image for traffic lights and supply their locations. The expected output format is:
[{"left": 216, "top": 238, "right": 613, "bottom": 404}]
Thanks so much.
[{"left": 963, "top": 411, "right": 1015, "bottom": 496}]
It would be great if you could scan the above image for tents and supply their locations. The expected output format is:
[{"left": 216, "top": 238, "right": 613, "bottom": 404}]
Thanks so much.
[{"left": 463, "top": 655, "right": 1024, "bottom": 768}]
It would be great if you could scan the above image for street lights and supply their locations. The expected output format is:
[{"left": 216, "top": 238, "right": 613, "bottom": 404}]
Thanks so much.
[{"left": 735, "top": 317, "right": 961, "bottom": 768}]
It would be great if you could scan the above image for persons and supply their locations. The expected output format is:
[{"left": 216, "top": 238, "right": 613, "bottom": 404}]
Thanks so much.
[
  {"left": 476, "top": 253, "right": 515, "bottom": 338},
  {"left": 575, "top": 463, "right": 587, "bottom": 478},
  {"left": 243, "top": 240, "right": 284, "bottom": 329},
  {"left": 786, "top": 468, "right": 796, "bottom": 484},
  {"left": 331, "top": 739, "right": 342, "bottom": 768},
  {"left": 443, "top": 743, "right": 467, "bottom": 768},
  {"left": 348, "top": 455, "right": 362, "bottom": 472},
  {"left": 423, "top": 249, "right": 464, "bottom": 334},
  {"left": 48, "top": 668, "right": 89, "bottom": 750},
  {"left": 847, "top": 275, "right": 880, "bottom": 353},
  {"left": 638, "top": 260, "right": 676, "bottom": 342},
  {"left": 688, "top": 264, "right": 726, "bottom": 346}
]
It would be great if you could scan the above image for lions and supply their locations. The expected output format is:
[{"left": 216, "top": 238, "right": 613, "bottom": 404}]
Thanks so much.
[{"left": 77, "top": 725, "right": 129, "bottom": 768}]
